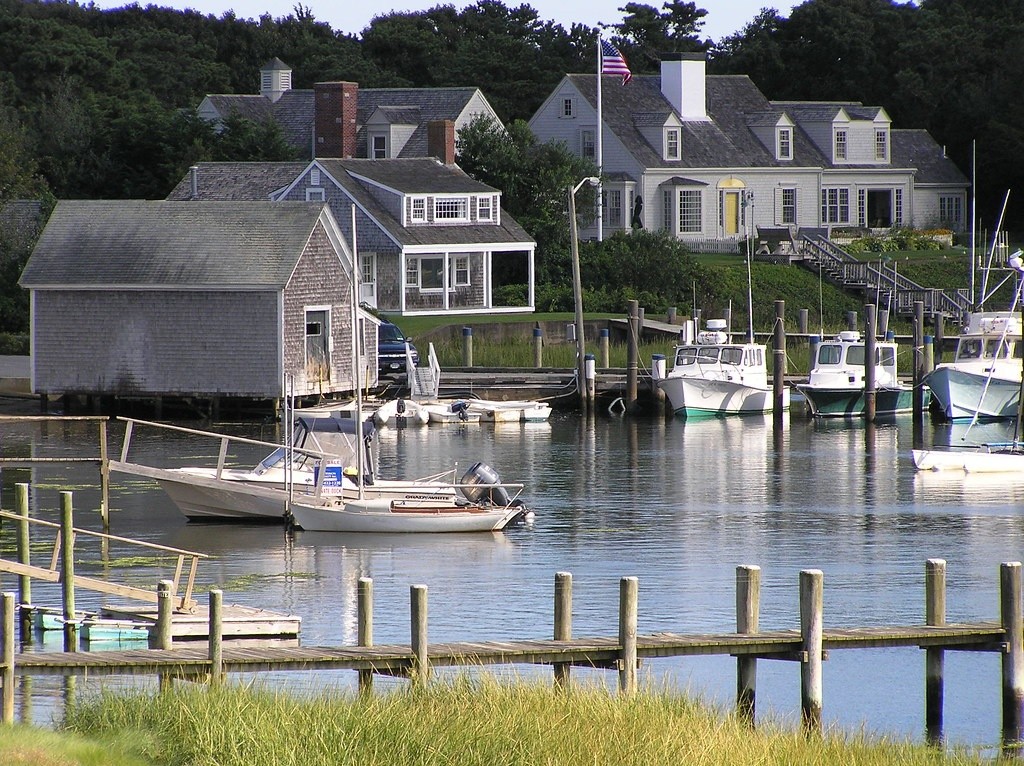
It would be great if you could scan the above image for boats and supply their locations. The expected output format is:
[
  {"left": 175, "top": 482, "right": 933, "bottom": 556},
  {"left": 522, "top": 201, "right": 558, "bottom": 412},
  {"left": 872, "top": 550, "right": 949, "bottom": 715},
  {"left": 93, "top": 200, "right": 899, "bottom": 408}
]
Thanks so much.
[
  {"left": 421, "top": 404, "right": 482, "bottom": 423},
  {"left": 795, "top": 281, "right": 932, "bottom": 420},
  {"left": 496, "top": 401, "right": 553, "bottom": 421},
  {"left": 329, "top": 364, "right": 388, "bottom": 423},
  {"left": 461, "top": 398, "right": 521, "bottom": 424},
  {"left": 153, "top": 372, "right": 463, "bottom": 525},
  {"left": 277, "top": 364, "right": 354, "bottom": 425},
  {"left": 654, "top": 236, "right": 792, "bottom": 423},
  {"left": 908, "top": 270, "right": 1024, "bottom": 470},
  {"left": 919, "top": 252, "right": 1024, "bottom": 424},
  {"left": 373, "top": 398, "right": 430, "bottom": 428}
]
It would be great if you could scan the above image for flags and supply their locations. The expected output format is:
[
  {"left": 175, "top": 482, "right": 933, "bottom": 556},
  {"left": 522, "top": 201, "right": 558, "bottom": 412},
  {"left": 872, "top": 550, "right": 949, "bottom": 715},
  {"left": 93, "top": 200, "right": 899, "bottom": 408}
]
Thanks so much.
[{"left": 600, "top": 38, "right": 631, "bottom": 87}]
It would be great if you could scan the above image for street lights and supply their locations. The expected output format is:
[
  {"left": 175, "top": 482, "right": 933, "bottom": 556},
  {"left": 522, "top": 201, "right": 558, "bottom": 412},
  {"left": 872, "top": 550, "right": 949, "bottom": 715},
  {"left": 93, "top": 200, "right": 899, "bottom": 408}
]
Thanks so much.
[{"left": 568, "top": 176, "right": 602, "bottom": 401}]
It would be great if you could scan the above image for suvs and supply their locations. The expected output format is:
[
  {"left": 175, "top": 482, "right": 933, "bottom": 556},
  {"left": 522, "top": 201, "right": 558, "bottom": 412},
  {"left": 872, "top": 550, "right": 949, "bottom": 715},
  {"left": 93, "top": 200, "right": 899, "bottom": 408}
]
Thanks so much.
[{"left": 379, "top": 320, "right": 419, "bottom": 376}]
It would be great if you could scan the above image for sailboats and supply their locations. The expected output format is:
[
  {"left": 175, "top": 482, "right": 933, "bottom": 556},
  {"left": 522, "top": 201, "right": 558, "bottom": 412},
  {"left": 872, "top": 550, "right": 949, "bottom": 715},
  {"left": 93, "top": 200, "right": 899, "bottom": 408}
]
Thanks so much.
[{"left": 291, "top": 204, "right": 537, "bottom": 534}]
